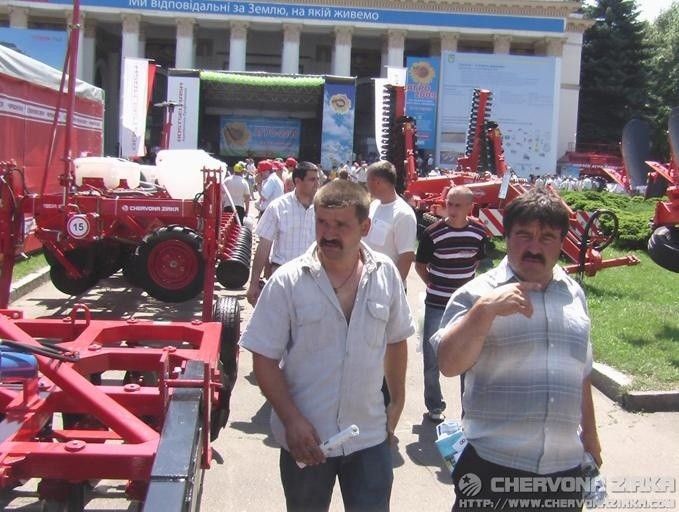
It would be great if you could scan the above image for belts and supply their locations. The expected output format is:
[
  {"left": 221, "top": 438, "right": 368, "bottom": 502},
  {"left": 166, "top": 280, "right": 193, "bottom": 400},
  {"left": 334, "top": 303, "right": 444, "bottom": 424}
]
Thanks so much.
[{"left": 271, "top": 262, "right": 280, "bottom": 267}]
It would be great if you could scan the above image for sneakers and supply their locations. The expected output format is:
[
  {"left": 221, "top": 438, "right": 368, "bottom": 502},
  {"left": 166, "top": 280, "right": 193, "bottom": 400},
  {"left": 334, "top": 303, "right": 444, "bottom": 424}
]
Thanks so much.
[{"left": 429, "top": 409, "right": 444, "bottom": 422}]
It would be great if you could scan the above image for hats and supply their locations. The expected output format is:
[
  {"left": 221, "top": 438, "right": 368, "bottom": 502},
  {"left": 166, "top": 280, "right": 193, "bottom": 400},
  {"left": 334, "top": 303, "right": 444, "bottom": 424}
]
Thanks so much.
[{"left": 234, "top": 158, "right": 297, "bottom": 174}]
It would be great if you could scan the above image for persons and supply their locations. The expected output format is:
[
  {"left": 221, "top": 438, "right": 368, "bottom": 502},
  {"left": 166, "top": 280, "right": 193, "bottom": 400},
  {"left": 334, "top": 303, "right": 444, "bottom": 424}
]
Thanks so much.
[
  {"left": 222, "top": 158, "right": 256, "bottom": 226},
  {"left": 254, "top": 161, "right": 286, "bottom": 217},
  {"left": 415, "top": 154, "right": 443, "bottom": 177},
  {"left": 238, "top": 179, "right": 417, "bottom": 512},
  {"left": 272, "top": 158, "right": 298, "bottom": 193},
  {"left": 415, "top": 185, "right": 489, "bottom": 422},
  {"left": 428, "top": 191, "right": 602, "bottom": 512},
  {"left": 247, "top": 161, "right": 319, "bottom": 309},
  {"left": 318, "top": 160, "right": 367, "bottom": 186},
  {"left": 529, "top": 174, "right": 593, "bottom": 192},
  {"left": 359, "top": 160, "right": 417, "bottom": 408}
]
